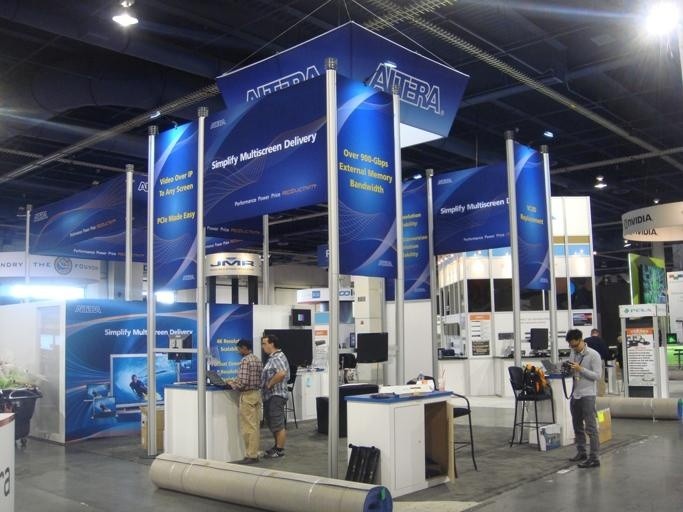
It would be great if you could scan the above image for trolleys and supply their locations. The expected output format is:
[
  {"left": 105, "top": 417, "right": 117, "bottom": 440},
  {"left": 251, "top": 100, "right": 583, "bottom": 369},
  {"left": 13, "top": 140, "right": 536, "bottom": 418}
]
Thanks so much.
[{"left": 0, "top": 387, "right": 43, "bottom": 449}]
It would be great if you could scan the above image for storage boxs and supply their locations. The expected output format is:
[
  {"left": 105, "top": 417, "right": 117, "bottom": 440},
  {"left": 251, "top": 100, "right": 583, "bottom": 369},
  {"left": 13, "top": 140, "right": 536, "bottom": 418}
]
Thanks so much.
[
  {"left": 583, "top": 408, "right": 613, "bottom": 444},
  {"left": 139, "top": 406, "right": 164, "bottom": 450}
]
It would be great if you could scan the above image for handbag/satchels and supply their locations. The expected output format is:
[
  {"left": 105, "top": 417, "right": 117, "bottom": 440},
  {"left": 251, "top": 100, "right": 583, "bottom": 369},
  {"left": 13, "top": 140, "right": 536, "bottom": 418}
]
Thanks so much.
[{"left": 522, "top": 364, "right": 546, "bottom": 394}]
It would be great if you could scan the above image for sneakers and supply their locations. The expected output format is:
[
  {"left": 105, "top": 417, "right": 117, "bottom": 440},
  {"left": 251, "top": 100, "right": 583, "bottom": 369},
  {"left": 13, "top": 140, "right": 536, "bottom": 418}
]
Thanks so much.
[
  {"left": 240, "top": 458, "right": 256, "bottom": 464},
  {"left": 265, "top": 447, "right": 284, "bottom": 458}
]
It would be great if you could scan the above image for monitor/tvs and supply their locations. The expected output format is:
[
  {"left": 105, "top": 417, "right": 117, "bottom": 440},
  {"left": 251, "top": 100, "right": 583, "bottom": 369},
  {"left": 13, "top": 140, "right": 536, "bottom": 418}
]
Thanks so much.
[
  {"left": 263, "top": 329, "right": 313, "bottom": 371},
  {"left": 169, "top": 335, "right": 191, "bottom": 361},
  {"left": 350, "top": 332, "right": 355, "bottom": 347},
  {"left": 110, "top": 354, "right": 179, "bottom": 416},
  {"left": 84, "top": 383, "right": 110, "bottom": 402},
  {"left": 93, "top": 398, "right": 117, "bottom": 418},
  {"left": 292, "top": 309, "right": 311, "bottom": 326},
  {"left": 357, "top": 332, "right": 388, "bottom": 363},
  {"left": 531, "top": 329, "right": 548, "bottom": 355},
  {"left": 666, "top": 333, "right": 677, "bottom": 346}
]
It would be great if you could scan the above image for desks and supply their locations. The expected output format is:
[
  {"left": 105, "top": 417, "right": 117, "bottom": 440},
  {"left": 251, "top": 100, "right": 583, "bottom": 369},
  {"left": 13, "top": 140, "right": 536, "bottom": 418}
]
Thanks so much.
[{"left": 437, "top": 355, "right": 550, "bottom": 396}]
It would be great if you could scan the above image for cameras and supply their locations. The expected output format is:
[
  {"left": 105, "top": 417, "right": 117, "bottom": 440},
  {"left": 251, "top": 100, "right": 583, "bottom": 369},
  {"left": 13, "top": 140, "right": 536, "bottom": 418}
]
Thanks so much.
[{"left": 562, "top": 360, "right": 577, "bottom": 371}]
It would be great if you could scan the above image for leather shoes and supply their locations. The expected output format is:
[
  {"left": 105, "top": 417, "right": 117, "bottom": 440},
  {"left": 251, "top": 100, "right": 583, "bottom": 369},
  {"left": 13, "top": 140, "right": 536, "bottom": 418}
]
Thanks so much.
[
  {"left": 570, "top": 454, "right": 587, "bottom": 461},
  {"left": 578, "top": 460, "right": 600, "bottom": 468}
]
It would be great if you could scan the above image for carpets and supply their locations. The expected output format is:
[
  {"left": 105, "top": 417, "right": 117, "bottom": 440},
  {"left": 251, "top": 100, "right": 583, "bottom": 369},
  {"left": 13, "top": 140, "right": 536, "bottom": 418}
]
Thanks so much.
[
  {"left": 150, "top": 453, "right": 393, "bottom": 512},
  {"left": 595, "top": 397, "right": 683, "bottom": 420}
]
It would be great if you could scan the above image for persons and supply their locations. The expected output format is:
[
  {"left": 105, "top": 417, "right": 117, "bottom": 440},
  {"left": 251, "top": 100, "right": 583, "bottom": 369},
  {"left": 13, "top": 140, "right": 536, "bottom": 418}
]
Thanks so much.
[
  {"left": 261, "top": 336, "right": 291, "bottom": 459},
  {"left": 100, "top": 402, "right": 112, "bottom": 412},
  {"left": 560, "top": 329, "right": 603, "bottom": 468},
  {"left": 92, "top": 389, "right": 102, "bottom": 399},
  {"left": 224, "top": 339, "right": 263, "bottom": 465},
  {"left": 130, "top": 375, "right": 147, "bottom": 399},
  {"left": 612, "top": 335, "right": 624, "bottom": 383},
  {"left": 583, "top": 328, "right": 611, "bottom": 396}
]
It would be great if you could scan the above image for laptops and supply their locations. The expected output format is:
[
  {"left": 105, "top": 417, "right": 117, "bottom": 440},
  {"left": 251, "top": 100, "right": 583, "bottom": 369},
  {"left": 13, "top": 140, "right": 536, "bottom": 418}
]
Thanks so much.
[{"left": 208, "top": 372, "right": 234, "bottom": 387}]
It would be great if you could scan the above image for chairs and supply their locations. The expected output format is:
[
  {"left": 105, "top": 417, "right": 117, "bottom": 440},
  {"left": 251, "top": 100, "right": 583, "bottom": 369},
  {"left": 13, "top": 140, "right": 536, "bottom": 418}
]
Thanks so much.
[
  {"left": 283, "top": 369, "right": 298, "bottom": 429},
  {"left": 316, "top": 384, "right": 378, "bottom": 437},
  {"left": 509, "top": 367, "right": 555, "bottom": 451},
  {"left": 407, "top": 376, "right": 477, "bottom": 479},
  {"left": 602, "top": 348, "right": 621, "bottom": 396}
]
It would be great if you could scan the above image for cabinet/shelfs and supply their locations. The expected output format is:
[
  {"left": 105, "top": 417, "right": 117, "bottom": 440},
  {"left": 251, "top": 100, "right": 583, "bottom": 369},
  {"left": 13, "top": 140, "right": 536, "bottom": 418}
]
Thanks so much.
[
  {"left": 288, "top": 368, "right": 329, "bottom": 420},
  {"left": 529, "top": 374, "right": 576, "bottom": 447},
  {"left": 344, "top": 391, "right": 453, "bottom": 499},
  {"left": 164, "top": 384, "right": 243, "bottom": 462}
]
